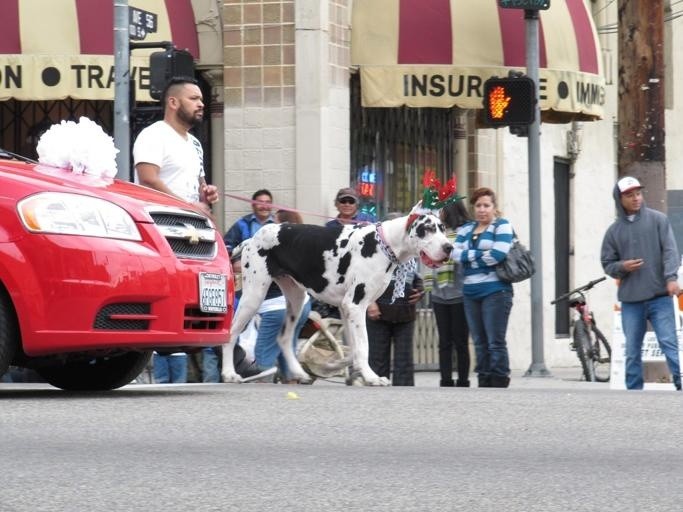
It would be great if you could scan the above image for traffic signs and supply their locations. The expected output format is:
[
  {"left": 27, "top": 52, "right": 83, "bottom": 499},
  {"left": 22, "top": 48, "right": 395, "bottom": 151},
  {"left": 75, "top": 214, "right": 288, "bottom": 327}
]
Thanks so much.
[{"left": 126, "top": 5, "right": 158, "bottom": 40}]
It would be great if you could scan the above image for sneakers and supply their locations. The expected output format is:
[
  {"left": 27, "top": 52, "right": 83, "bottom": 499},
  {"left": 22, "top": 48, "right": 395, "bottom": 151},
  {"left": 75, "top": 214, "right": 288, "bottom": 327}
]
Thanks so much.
[{"left": 236, "top": 356, "right": 278, "bottom": 381}]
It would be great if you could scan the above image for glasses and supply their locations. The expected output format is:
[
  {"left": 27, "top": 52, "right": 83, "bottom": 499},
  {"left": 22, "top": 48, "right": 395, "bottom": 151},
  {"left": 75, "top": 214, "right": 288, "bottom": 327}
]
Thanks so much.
[{"left": 340, "top": 199, "right": 355, "bottom": 204}]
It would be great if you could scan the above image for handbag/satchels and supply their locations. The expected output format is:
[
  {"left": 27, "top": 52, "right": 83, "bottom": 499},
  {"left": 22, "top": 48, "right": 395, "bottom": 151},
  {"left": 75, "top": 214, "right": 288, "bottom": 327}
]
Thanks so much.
[{"left": 493, "top": 219, "right": 536, "bottom": 283}]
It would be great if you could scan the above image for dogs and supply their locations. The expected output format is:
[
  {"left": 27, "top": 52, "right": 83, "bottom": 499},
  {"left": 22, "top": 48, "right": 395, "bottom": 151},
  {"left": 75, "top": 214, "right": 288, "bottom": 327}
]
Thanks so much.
[{"left": 221, "top": 200, "right": 453, "bottom": 388}]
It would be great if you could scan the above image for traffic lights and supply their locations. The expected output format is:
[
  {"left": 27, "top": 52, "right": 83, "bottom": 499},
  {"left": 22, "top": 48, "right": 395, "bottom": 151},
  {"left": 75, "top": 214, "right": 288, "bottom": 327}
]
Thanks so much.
[{"left": 481, "top": 76, "right": 536, "bottom": 130}]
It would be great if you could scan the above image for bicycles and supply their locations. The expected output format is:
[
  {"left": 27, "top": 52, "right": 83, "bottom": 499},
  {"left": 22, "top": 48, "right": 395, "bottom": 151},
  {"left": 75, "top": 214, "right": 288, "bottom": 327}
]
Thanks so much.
[{"left": 548, "top": 275, "right": 613, "bottom": 384}]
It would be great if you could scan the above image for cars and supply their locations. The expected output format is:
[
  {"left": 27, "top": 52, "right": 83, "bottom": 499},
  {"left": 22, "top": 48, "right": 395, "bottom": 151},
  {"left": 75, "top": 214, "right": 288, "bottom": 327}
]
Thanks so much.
[{"left": 0, "top": 148, "right": 236, "bottom": 391}]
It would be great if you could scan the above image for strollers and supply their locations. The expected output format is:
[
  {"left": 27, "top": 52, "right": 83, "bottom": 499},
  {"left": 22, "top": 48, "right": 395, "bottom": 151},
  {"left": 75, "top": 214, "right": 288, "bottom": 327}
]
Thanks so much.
[{"left": 273, "top": 288, "right": 358, "bottom": 385}]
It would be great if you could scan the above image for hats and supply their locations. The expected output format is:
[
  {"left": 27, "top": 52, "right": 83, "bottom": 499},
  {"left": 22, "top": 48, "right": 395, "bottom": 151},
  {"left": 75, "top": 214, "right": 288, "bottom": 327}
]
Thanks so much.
[
  {"left": 616, "top": 176, "right": 645, "bottom": 193},
  {"left": 337, "top": 188, "right": 360, "bottom": 204}
]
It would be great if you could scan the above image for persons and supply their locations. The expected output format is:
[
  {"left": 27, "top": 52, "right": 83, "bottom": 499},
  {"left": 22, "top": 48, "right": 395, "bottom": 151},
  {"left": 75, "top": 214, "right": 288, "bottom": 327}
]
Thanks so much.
[
  {"left": 420, "top": 199, "right": 472, "bottom": 387},
  {"left": 223, "top": 188, "right": 274, "bottom": 272},
  {"left": 450, "top": 188, "right": 516, "bottom": 388},
  {"left": 325, "top": 188, "right": 378, "bottom": 227},
  {"left": 132, "top": 77, "right": 218, "bottom": 382},
  {"left": 255, "top": 209, "right": 312, "bottom": 382},
  {"left": 367, "top": 212, "right": 424, "bottom": 387},
  {"left": 600, "top": 176, "right": 682, "bottom": 390}
]
